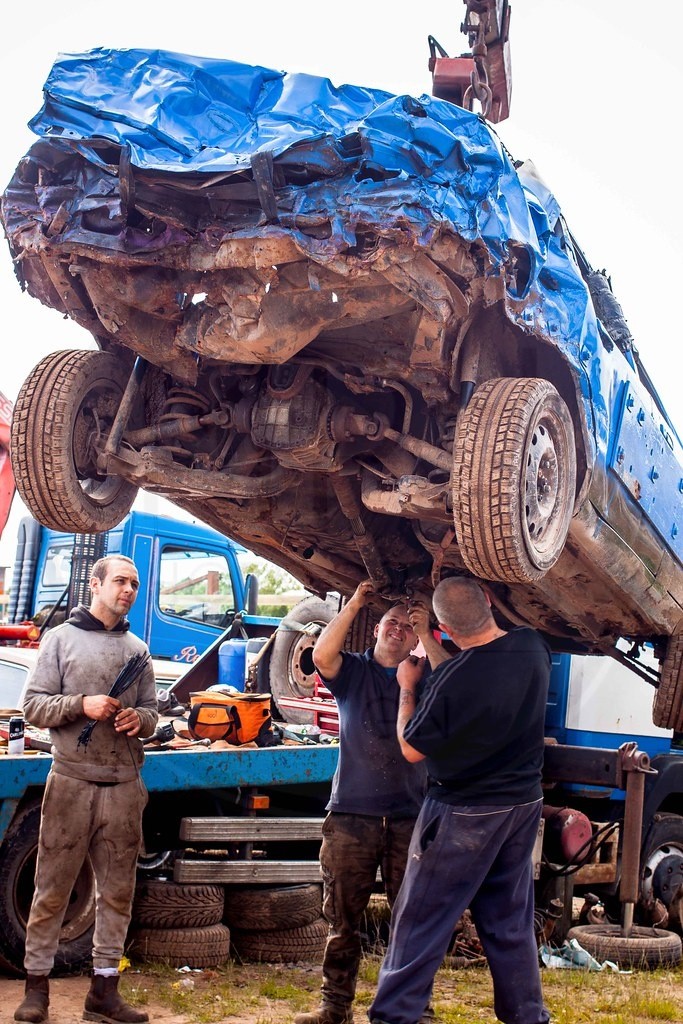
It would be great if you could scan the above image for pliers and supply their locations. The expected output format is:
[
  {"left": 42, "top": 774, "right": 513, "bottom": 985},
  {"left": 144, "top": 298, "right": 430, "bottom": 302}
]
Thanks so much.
[{"left": 404, "top": 595, "right": 415, "bottom": 610}]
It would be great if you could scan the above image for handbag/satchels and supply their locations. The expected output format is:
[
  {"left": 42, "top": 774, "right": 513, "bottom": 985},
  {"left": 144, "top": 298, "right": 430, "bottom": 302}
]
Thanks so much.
[{"left": 188, "top": 689, "right": 284, "bottom": 748}]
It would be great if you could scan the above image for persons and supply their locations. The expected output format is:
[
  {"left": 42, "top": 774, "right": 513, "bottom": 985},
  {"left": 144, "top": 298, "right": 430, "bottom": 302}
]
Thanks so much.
[
  {"left": 367, "top": 575, "right": 552, "bottom": 1024},
  {"left": 14, "top": 554, "right": 158, "bottom": 1024},
  {"left": 293, "top": 578, "right": 450, "bottom": 1024}
]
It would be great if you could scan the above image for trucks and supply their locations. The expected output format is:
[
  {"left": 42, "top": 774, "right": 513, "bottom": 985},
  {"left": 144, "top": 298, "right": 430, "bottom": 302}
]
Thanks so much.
[
  {"left": 0, "top": 508, "right": 259, "bottom": 705},
  {"left": 0, "top": 586, "right": 683, "bottom": 978}
]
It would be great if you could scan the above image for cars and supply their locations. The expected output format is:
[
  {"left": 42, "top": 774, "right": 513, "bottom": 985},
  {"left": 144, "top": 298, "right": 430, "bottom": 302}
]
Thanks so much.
[{"left": 6, "top": 47, "right": 683, "bottom": 728}]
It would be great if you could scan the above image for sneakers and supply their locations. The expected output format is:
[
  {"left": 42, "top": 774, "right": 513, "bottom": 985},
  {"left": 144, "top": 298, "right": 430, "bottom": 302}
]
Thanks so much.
[
  {"left": 157, "top": 689, "right": 171, "bottom": 714},
  {"left": 422, "top": 1005, "right": 435, "bottom": 1019},
  {"left": 164, "top": 694, "right": 185, "bottom": 716},
  {"left": 294, "top": 1007, "right": 354, "bottom": 1024}
]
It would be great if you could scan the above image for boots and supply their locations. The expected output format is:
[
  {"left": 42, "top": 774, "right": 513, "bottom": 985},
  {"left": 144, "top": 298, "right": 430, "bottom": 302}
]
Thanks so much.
[
  {"left": 14, "top": 975, "right": 50, "bottom": 1024},
  {"left": 83, "top": 968, "right": 151, "bottom": 1024}
]
